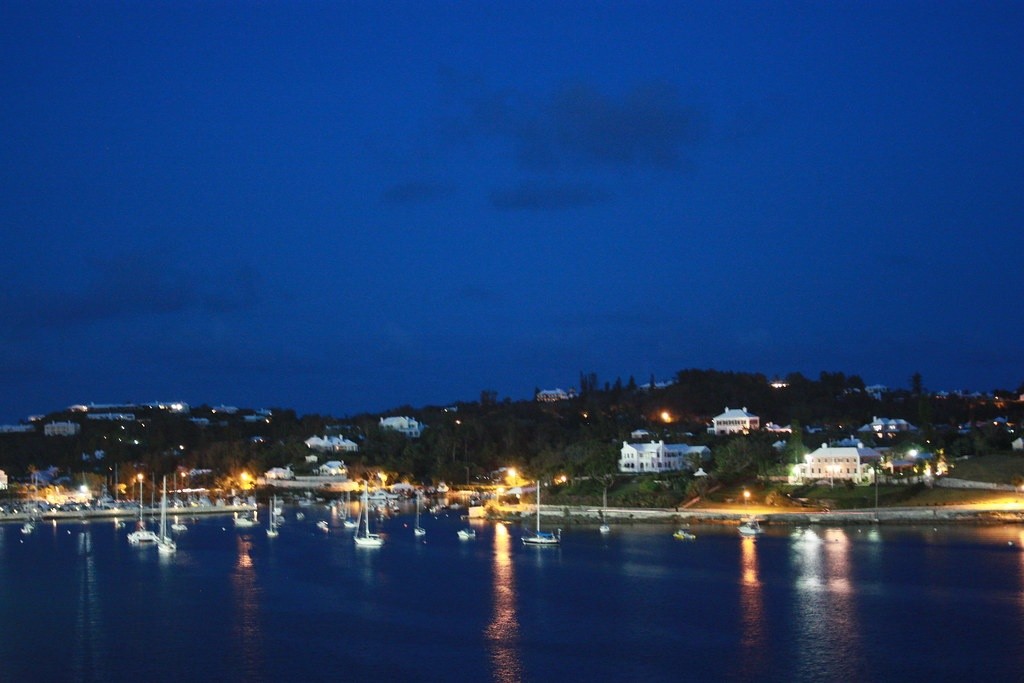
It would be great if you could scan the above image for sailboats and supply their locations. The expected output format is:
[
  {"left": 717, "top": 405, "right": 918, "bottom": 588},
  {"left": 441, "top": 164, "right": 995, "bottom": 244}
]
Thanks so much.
[
  {"left": 520, "top": 481, "right": 561, "bottom": 544},
  {"left": 352, "top": 481, "right": 383, "bottom": 548},
  {"left": 129, "top": 477, "right": 160, "bottom": 548},
  {"left": 412, "top": 495, "right": 425, "bottom": 537},
  {"left": 157, "top": 476, "right": 176, "bottom": 556}
]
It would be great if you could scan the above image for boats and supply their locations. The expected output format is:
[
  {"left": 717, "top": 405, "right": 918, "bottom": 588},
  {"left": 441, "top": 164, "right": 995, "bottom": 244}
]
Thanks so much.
[
  {"left": 673, "top": 529, "right": 696, "bottom": 540},
  {"left": 457, "top": 529, "right": 476, "bottom": 538},
  {"left": 738, "top": 520, "right": 765, "bottom": 535},
  {"left": 235, "top": 494, "right": 331, "bottom": 539}
]
